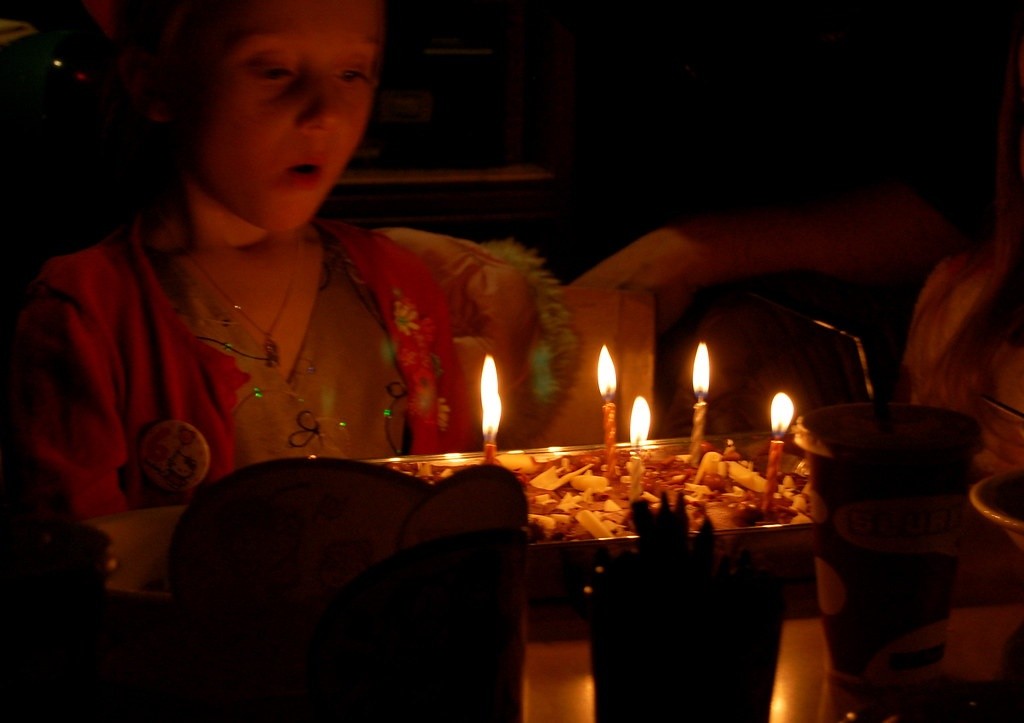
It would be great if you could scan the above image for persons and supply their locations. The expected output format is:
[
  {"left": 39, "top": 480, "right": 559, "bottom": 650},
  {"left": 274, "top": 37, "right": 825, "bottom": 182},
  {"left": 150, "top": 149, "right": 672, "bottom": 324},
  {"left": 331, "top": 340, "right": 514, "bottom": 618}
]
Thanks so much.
[
  {"left": 0, "top": 1, "right": 590, "bottom": 529},
  {"left": 618, "top": 0, "right": 1024, "bottom": 485}
]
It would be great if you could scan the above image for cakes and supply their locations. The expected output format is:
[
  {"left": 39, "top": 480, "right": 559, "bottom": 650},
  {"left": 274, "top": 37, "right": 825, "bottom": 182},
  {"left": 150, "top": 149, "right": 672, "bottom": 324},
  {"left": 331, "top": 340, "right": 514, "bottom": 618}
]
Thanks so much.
[{"left": 363, "top": 429, "right": 816, "bottom": 599}]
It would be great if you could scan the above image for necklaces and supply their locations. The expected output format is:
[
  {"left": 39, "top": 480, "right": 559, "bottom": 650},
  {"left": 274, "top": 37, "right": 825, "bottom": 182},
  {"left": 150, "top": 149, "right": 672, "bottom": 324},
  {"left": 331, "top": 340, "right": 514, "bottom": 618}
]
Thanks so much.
[{"left": 150, "top": 205, "right": 303, "bottom": 369}]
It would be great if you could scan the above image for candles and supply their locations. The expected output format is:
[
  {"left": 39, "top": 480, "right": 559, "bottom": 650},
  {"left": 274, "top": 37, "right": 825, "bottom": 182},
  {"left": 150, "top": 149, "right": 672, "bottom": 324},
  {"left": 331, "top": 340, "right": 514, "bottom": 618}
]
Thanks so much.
[
  {"left": 597, "top": 345, "right": 621, "bottom": 479},
  {"left": 480, "top": 354, "right": 502, "bottom": 464},
  {"left": 761, "top": 392, "right": 794, "bottom": 513},
  {"left": 688, "top": 342, "right": 710, "bottom": 467},
  {"left": 630, "top": 396, "right": 651, "bottom": 512}
]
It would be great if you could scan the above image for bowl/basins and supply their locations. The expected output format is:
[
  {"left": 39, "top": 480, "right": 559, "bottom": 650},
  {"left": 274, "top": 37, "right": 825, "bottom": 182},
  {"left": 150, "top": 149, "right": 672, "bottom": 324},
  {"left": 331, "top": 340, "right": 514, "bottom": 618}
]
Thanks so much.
[{"left": 969, "top": 466, "right": 1024, "bottom": 553}]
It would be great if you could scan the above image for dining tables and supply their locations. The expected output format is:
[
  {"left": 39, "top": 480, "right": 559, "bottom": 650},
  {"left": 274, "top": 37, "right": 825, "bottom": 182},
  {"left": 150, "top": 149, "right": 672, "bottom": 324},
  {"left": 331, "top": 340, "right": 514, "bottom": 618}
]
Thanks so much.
[{"left": 74, "top": 471, "right": 1024, "bottom": 722}]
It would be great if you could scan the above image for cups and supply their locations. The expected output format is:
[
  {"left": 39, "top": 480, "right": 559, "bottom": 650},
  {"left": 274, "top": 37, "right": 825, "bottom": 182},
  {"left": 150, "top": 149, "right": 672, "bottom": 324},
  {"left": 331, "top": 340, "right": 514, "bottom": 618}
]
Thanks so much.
[{"left": 791, "top": 404, "right": 981, "bottom": 688}]
